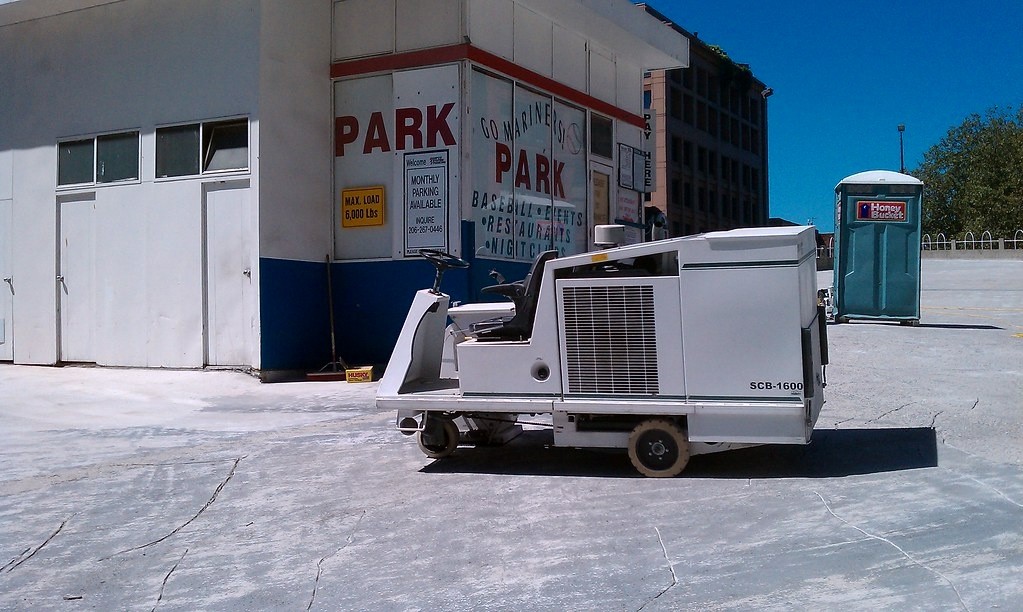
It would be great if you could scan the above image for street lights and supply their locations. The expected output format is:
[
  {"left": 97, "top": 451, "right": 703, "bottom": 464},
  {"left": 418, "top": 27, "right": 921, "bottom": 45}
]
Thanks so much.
[{"left": 897, "top": 124, "right": 905, "bottom": 174}]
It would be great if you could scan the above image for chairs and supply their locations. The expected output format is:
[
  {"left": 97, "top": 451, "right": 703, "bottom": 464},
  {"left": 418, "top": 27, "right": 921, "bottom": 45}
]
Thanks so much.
[{"left": 479, "top": 249, "right": 560, "bottom": 341}]
